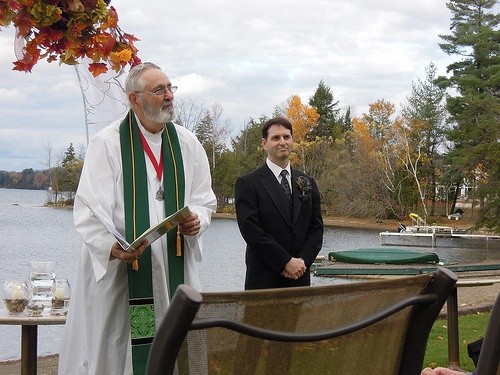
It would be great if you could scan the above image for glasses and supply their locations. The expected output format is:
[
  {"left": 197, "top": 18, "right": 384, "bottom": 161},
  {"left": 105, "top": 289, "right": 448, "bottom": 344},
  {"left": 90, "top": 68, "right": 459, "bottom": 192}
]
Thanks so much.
[{"left": 135, "top": 86, "right": 178, "bottom": 98}]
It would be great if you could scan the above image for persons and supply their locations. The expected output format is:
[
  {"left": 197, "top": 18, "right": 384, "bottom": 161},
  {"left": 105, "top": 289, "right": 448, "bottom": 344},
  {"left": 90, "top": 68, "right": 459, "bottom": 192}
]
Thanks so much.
[
  {"left": 419, "top": 287, "right": 500, "bottom": 375},
  {"left": 58, "top": 62, "right": 217, "bottom": 374},
  {"left": 228, "top": 116, "right": 324, "bottom": 375}
]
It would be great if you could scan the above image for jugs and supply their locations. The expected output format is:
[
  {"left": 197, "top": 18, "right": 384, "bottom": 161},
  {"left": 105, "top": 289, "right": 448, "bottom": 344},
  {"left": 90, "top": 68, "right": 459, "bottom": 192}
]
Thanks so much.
[{"left": 27, "top": 261, "right": 57, "bottom": 317}]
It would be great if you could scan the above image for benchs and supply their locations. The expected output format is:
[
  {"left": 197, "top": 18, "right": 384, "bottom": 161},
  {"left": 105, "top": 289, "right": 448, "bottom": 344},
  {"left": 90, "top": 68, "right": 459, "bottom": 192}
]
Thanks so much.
[{"left": 145, "top": 265, "right": 456, "bottom": 375}]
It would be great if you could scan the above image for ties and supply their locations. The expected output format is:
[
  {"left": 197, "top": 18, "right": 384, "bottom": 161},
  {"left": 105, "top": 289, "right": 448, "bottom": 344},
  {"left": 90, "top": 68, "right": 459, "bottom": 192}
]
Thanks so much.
[{"left": 280, "top": 170, "right": 293, "bottom": 218}]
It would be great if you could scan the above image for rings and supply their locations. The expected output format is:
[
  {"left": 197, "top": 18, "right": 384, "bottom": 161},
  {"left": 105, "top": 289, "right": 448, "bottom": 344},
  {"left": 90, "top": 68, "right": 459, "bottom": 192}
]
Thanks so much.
[{"left": 192, "top": 226, "right": 196, "bottom": 232}]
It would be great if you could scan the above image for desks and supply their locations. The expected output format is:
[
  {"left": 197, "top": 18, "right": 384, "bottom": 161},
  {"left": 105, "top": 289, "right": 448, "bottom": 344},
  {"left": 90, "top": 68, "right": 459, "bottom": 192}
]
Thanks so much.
[{"left": 0, "top": 304, "right": 71, "bottom": 375}]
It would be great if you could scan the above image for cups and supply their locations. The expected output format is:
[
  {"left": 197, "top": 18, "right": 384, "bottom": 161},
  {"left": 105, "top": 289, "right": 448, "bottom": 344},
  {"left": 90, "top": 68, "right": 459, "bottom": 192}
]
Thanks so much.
[
  {"left": 51, "top": 279, "right": 71, "bottom": 315},
  {"left": 3, "top": 280, "right": 29, "bottom": 317}
]
